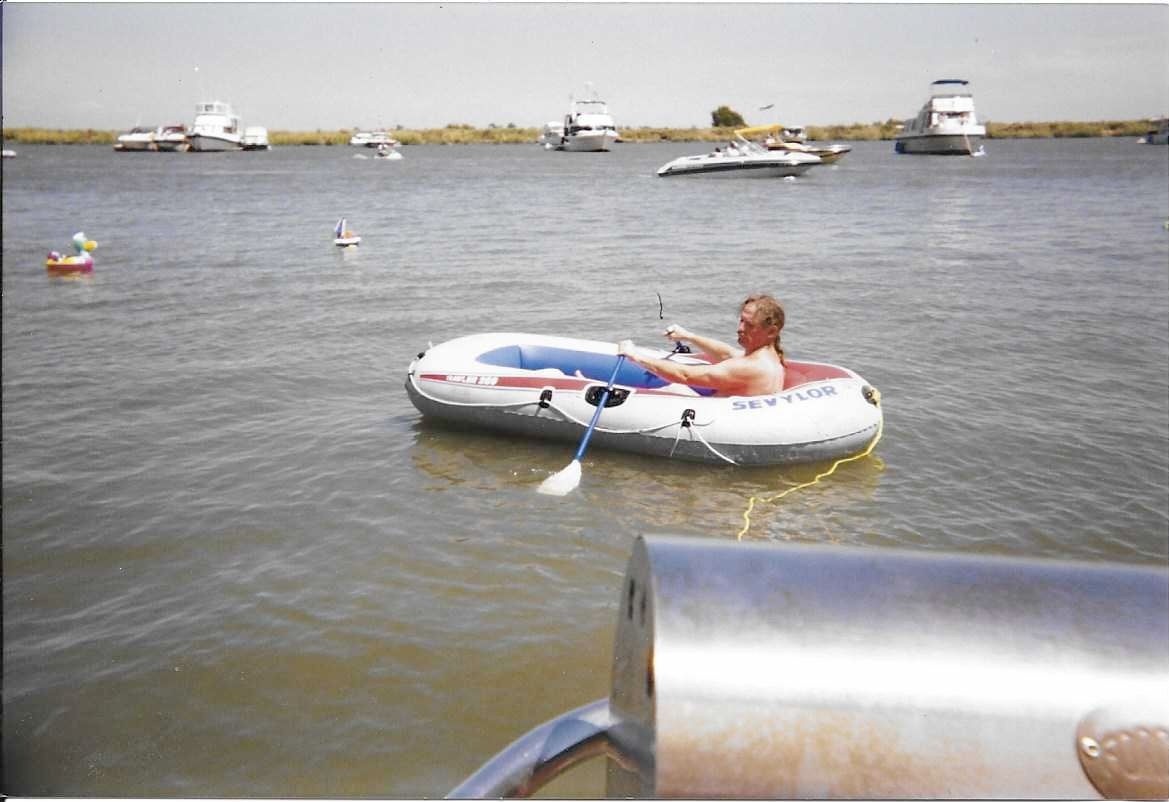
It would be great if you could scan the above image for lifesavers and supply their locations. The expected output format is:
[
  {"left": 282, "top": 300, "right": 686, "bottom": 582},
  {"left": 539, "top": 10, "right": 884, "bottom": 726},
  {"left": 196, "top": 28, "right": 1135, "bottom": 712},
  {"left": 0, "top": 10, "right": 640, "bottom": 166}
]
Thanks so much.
[{"left": 46, "top": 231, "right": 99, "bottom": 277}]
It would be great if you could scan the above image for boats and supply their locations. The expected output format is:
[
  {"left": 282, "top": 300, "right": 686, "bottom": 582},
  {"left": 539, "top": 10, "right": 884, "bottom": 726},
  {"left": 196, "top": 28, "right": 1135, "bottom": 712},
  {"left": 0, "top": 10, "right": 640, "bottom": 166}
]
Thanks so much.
[
  {"left": 46, "top": 255, "right": 94, "bottom": 278},
  {"left": 765, "top": 143, "right": 852, "bottom": 163},
  {"left": 349, "top": 132, "right": 397, "bottom": 146},
  {"left": 657, "top": 147, "right": 820, "bottom": 178},
  {"left": 242, "top": 128, "right": 269, "bottom": 149},
  {"left": 153, "top": 126, "right": 190, "bottom": 152},
  {"left": 558, "top": 112, "right": 620, "bottom": 152},
  {"left": 335, "top": 237, "right": 361, "bottom": 246},
  {"left": 374, "top": 150, "right": 402, "bottom": 160},
  {"left": 894, "top": 95, "right": 986, "bottom": 154},
  {"left": 405, "top": 331, "right": 884, "bottom": 467},
  {"left": 118, "top": 133, "right": 156, "bottom": 149},
  {"left": 186, "top": 102, "right": 240, "bottom": 152},
  {"left": 544, "top": 130, "right": 564, "bottom": 145}
]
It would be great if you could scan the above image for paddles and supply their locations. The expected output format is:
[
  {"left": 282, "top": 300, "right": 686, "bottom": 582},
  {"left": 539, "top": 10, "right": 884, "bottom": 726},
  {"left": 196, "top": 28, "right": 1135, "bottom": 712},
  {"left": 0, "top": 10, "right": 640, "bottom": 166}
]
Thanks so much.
[
  {"left": 533, "top": 349, "right": 633, "bottom": 498},
  {"left": 664, "top": 326, "right": 696, "bottom": 358}
]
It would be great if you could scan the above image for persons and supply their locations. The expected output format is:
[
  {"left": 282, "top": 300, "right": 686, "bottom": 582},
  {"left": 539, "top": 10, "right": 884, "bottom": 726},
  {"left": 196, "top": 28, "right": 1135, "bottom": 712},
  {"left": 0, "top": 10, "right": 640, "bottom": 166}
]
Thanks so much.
[{"left": 616, "top": 294, "right": 787, "bottom": 397}]
